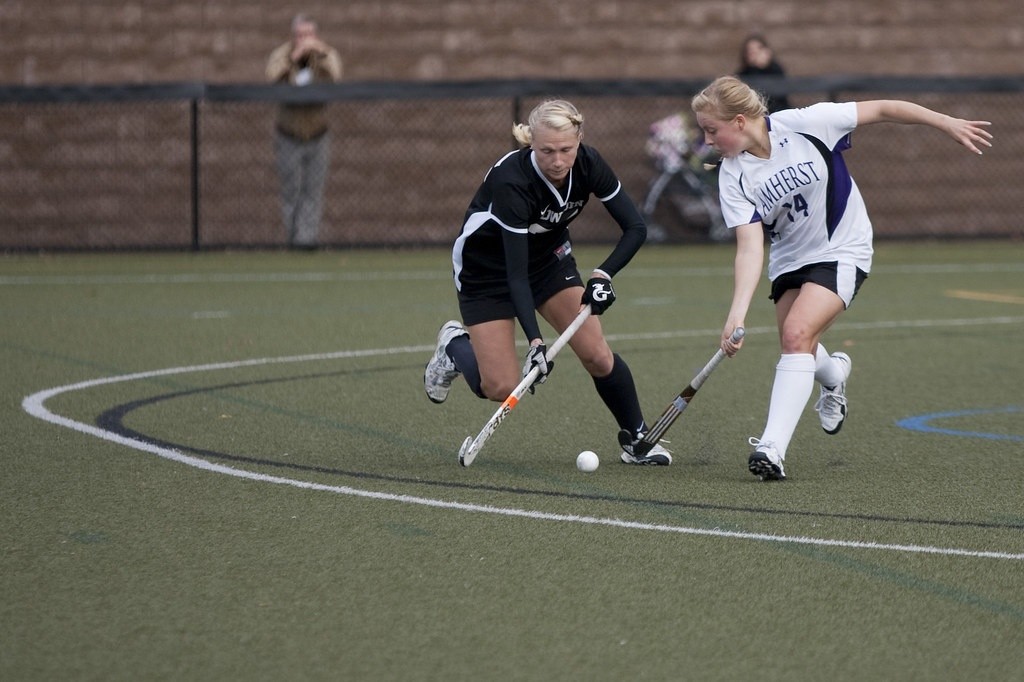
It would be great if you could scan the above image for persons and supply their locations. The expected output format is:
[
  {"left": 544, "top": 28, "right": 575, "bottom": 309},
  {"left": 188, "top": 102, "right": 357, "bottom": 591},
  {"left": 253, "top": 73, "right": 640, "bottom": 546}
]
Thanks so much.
[
  {"left": 692, "top": 74, "right": 994, "bottom": 481},
  {"left": 734, "top": 34, "right": 793, "bottom": 113},
  {"left": 424, "top": 100, "right": 673, "bottom": 467},
  {"left": 265, "top": 12, "right": 343, "bottom": 250}
]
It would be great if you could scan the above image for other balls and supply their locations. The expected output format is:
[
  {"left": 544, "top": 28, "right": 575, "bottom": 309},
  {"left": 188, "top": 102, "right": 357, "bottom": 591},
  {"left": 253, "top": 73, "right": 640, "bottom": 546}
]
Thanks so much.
[{"left": 576, "top": 449, "right": 600, "bottom": 473}]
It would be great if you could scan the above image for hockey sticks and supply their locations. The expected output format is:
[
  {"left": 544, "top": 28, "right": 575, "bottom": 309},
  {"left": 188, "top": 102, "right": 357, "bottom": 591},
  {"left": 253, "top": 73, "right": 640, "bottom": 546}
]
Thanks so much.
[
  {"left": 455, "top": 302, "right": 592, "bottom": 469},
  {"left": 616, "top": 325, "right": 747, "bottom": 460}
]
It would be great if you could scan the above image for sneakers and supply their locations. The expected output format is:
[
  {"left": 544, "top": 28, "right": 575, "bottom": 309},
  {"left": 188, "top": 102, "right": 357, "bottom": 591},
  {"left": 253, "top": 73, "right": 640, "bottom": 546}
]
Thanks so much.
[
  {"left": 424, "top": 320, "right": 470, "bottom": 404},
  {"left": 621, "top": 424, "right": 673, "bottom": 467},
  {"left": 748, "top": 437, "right": 787, "bottom": 481},
  {"left": 814, "top": 352, "right": 852, "bottom": 435}
]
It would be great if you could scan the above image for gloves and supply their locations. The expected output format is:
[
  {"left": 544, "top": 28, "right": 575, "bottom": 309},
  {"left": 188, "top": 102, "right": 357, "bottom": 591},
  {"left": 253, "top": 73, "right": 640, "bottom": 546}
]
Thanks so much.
[{"left": 522, "top": 341, "right": 554, "bottom": 395}]
